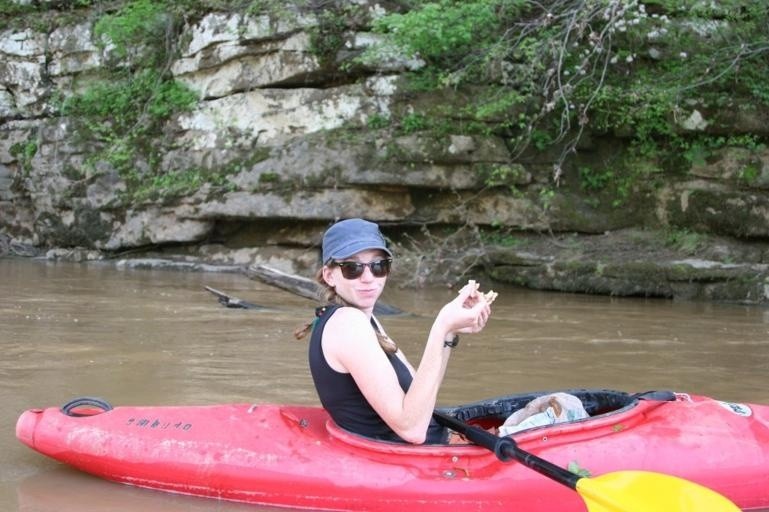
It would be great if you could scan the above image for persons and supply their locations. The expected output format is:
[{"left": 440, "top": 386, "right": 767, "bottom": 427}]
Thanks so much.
[{"left": 294, "top": 215, "right": 501, "bottom": 447}]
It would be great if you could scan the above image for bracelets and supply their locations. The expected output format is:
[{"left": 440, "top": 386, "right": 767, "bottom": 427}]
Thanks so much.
[{"left": 444, "top": 334, "right": 461, "bottom": 350}]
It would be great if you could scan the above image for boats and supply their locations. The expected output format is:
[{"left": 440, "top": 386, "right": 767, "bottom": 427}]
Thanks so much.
[{"left": 16, "top": 390, "right": 769, "bottom": 511}]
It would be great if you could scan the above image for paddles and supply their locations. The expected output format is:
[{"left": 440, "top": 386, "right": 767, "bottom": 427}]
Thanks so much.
[{"left": 434, "top": 410, "right": 739, "bottom": 512}]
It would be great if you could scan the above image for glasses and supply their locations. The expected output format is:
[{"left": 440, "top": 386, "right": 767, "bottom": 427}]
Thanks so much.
[{"left": 333, "top": 259, "right": 393, "bottom": 279}]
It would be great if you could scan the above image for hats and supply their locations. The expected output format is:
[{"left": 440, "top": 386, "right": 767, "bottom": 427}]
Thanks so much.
[{"left": 323, "top": 219, "right": 393, "bottom": 265}]
